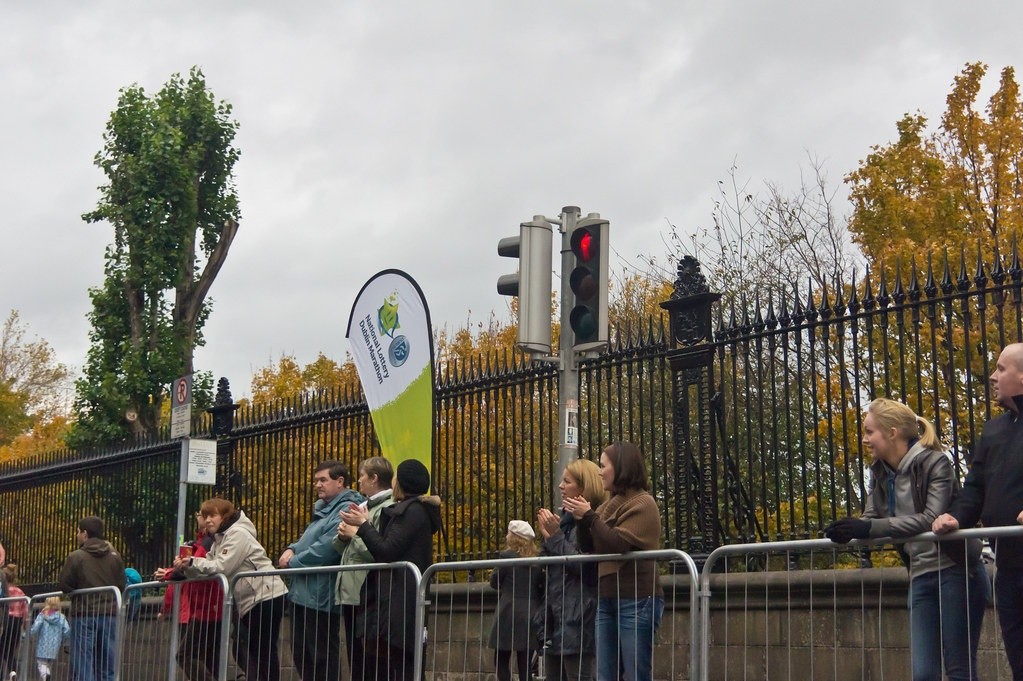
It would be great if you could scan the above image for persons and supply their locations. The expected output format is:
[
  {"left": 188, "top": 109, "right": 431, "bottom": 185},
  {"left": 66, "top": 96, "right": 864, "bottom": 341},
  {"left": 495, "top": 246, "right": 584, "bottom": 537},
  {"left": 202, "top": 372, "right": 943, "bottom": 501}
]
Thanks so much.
[
  {"left": 823, "top": 400, "right": 992, "bottom": 681},
  {"left": 491, "top": 520, "right": 540, "bottom": 681},
  {"left": 175, "top": 498, "right": 288, "bottom": 681},
  {"left": 155, "top": 510, "right": 224, "bottom": 681},
  {"left": 932, "top": 341, "right": 1023, "bottom": 681},
  {"left": 333, "top": 457, "right": 396, "bottom": 669},
  {"left": 562, "top": 439, "right": 664, "bottom": 681},
  {"left": 30, "top": 597, "right": 71, "bottom": 681},
  {"left": 0, "top": 543, "right": 9, "bottom": 681},
  {"left": 537, "top": 456, "right": 600, "bottom": 681},
  {"left": 156, "top": 541, "right": 198, "bottom": 644},
  {"left": 3, "top": 565, "right": 28, "bottom": 681},
  {"left": 279, "top": 460, "right": 365, "bottom": 681},
  {"left": 60, "top": 517, "right": 127, "bottom": 680},
  {"left": 340, "top": 460, "right": 443, "bottom": 681}
]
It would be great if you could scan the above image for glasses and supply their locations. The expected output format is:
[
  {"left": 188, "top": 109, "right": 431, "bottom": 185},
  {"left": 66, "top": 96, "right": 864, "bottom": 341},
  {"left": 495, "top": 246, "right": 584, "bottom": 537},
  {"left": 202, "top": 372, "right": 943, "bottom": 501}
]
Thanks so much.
[{"left": 195, "top": 512, "right": 203, "bottom": 519}]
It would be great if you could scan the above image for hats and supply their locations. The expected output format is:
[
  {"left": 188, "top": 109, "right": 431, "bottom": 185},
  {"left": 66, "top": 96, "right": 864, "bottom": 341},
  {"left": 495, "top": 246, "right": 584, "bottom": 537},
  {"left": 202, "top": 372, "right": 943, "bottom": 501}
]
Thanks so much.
[
  {"left": 507, "top": 520, "right": 535, "bottom": 541},
  {"left": 396, "top": 459, "right": 430, "bottom": 495}
]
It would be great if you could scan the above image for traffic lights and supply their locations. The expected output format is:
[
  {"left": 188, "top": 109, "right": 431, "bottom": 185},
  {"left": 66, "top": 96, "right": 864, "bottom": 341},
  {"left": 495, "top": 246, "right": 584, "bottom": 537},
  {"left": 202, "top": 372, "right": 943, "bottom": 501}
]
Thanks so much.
[
  {"left": 569, "top": 219, "right": 610, "bottom": 361},
  {"left": 497, "top": 215, "right": 553, "bottom": 354}
]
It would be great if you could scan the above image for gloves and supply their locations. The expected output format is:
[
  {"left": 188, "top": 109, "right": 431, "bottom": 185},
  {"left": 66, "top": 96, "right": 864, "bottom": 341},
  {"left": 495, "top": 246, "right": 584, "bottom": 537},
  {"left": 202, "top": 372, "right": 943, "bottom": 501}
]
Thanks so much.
[{"left": 824, "top": 516, "right": 871, "bottom": 544}]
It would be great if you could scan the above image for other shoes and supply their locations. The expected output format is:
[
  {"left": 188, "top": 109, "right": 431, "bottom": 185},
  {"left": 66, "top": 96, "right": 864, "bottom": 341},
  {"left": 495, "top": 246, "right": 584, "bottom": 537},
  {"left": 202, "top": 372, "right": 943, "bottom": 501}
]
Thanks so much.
[
  {"left": 42, "top": 674, "right": 50, "bottom": 681},
  {"left": 10, "top": 671, "right": 17, "bottom": 681}
]
